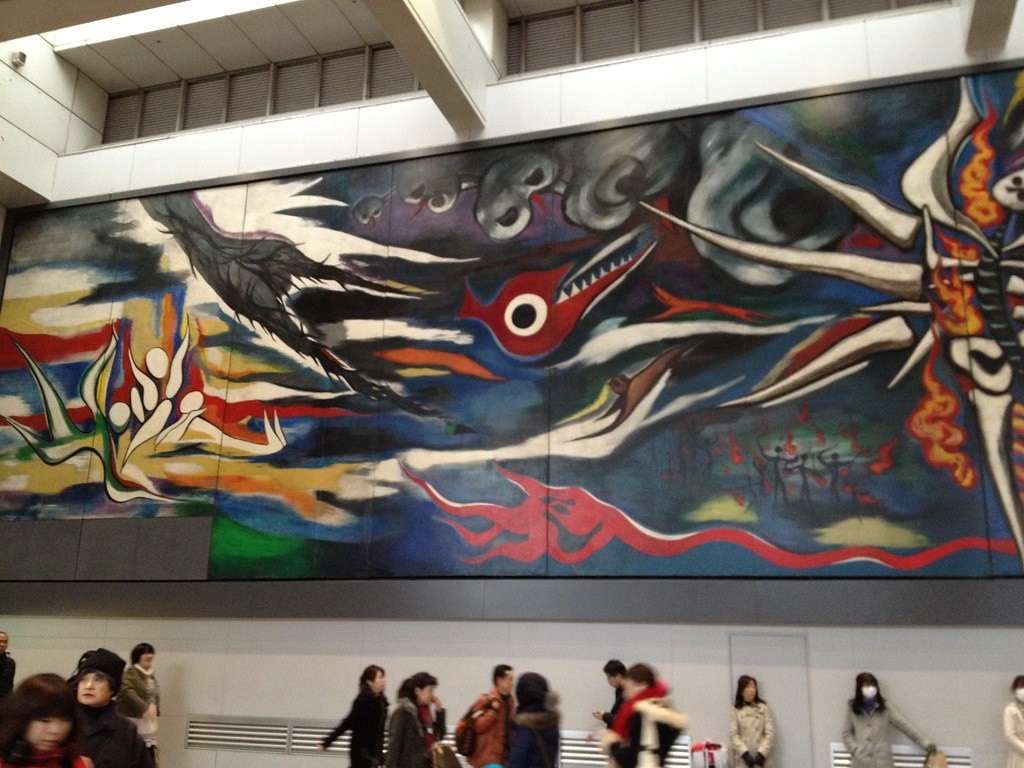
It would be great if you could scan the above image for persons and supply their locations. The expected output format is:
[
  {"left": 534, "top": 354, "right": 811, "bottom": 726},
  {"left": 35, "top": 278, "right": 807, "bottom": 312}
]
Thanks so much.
[
  {"left": 592, "top": 658, "right": 687, "bottom": 768},
  {"left": 0, "top": 631, "right": 161, "bottom": 768},
  {"left": 730, "top": 675, "right": 775, "bottom": 768},
  {"left": 1004, "top": 675, "right": 1024, "bottom": 768},
  {"left": 841, "top": 672, "right": 937, "bottom": 768},
  {"left": 320, "top": 665, "right": 562, "bottom": 768}
]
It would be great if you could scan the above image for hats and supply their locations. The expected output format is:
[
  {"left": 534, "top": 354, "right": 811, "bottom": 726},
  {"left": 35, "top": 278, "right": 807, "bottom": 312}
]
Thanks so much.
[{"left": 78, "top": 648, "right": 127, "bottom": 697}]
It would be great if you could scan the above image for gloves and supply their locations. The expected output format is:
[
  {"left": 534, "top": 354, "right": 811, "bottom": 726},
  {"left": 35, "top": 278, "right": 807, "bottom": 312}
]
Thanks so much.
[
  {"left": 925, "top": 744, "right": 936, "bottom": 754},
  {"left": 741, "top": 750, "right": 755, "bottom": 768},
  {"left": 755, "top": 751, "right": 765, "bottom": 767}
]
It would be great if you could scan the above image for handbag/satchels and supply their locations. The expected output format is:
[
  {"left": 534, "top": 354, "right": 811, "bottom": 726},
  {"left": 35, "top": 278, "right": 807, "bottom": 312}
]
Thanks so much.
[
  {"left": 924, "top": 744, "right": 947, "bottom": 768},
  {"left": 691, "top": 738, "right": 729, "bottom": 768},
  {"left": 455, "top": 703, "right": 491, "bottom": 756}
]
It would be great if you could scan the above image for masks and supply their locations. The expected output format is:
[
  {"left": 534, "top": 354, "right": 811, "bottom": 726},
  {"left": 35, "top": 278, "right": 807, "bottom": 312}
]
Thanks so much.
[
  {"left": 1015, "top": 688, "right": 1024, "bottom": 702},
  {"left": 862, "top": 686, "right": 878, "bottom": 697}
]
